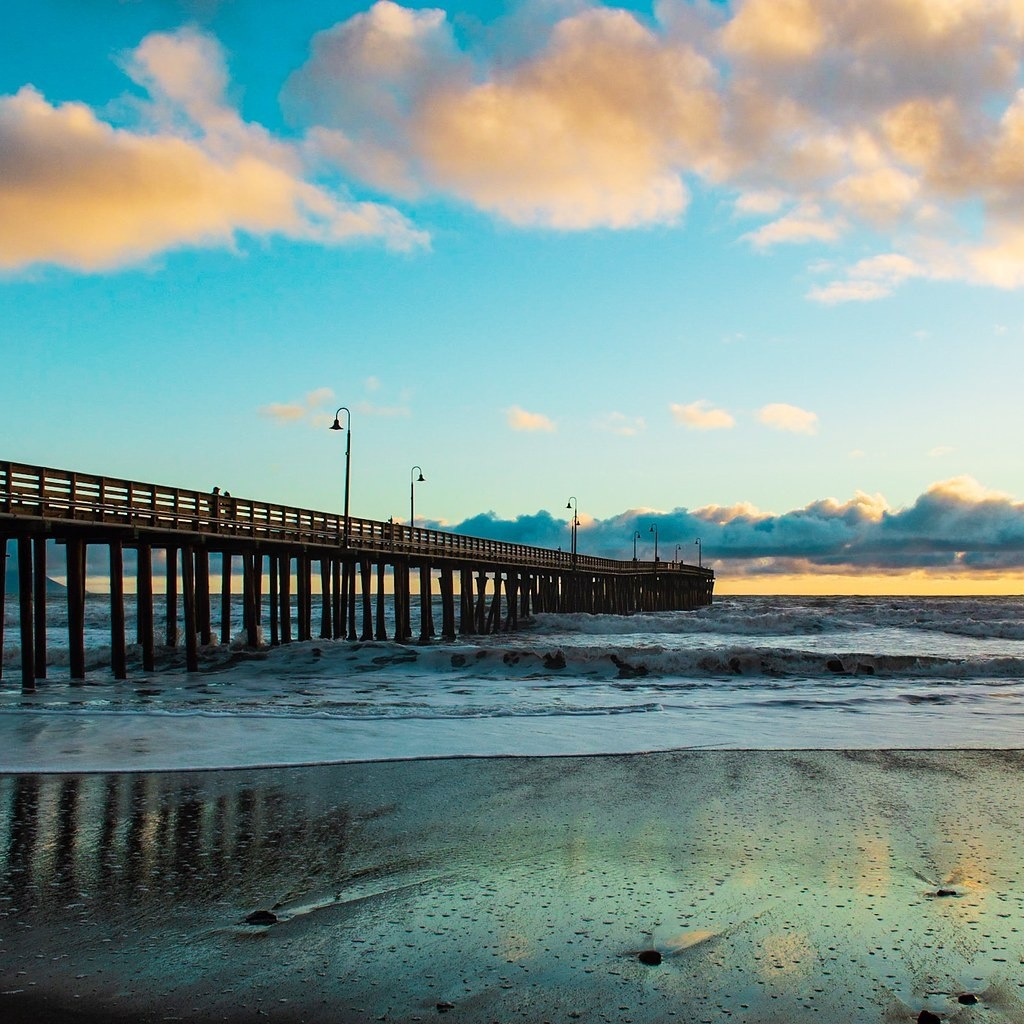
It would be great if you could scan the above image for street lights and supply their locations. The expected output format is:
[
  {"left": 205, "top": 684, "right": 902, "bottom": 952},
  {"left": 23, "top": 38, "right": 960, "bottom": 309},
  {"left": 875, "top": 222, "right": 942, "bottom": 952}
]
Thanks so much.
[
  {"left": 410, "top": 466, "right": 426, "bottom": 527},
  {"left": 695, "top": 537, "right": 701, "bottom": 568},
  {"left": 633, "top": 531, "right": 641, "bottom": 562},
  {"left": 328, "top": 406, "right": 351, "bottom": 547},
  {"left": 649, "top": 523, "right": 657, "bottom": 562},
  {"left": 675, "top": 544, "right": 681, "bottom": 563},
  {"left": 564, "top": 496, "right": 581, "bottom": 571}
]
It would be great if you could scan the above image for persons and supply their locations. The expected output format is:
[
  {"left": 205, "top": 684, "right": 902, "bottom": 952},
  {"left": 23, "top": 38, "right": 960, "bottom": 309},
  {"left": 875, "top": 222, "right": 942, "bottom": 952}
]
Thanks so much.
[
  {"left": 207, "top": 487, "right": 221, "bottom": 523},
  {"left": 224, "top": 491, "right": 236, "bottom": 528}
]
